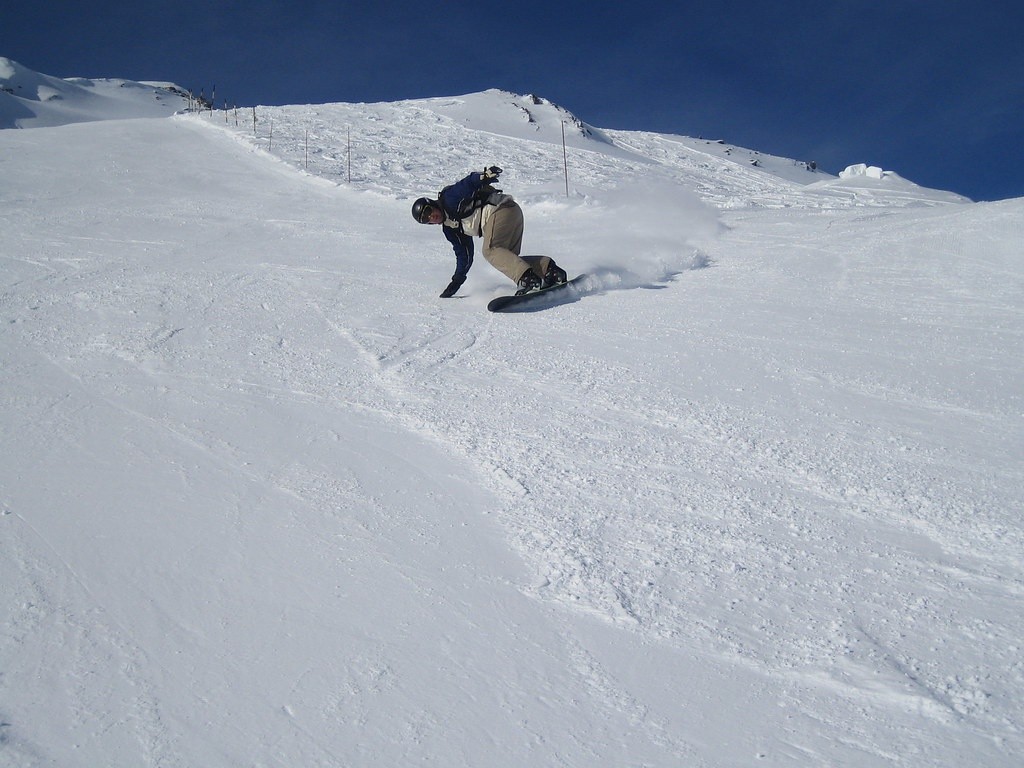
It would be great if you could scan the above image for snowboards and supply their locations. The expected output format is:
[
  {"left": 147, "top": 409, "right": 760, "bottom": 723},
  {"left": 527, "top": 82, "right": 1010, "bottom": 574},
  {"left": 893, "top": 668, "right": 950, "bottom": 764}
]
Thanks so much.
[{"left": 487, "top": 273, "right": 590, "bottom": 312}]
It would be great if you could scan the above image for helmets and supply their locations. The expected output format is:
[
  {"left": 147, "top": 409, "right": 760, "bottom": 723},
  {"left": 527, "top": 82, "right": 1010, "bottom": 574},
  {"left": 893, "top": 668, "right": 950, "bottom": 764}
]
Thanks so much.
[{"left": 411, "top": 197, "right": 435, "bottom": 226}]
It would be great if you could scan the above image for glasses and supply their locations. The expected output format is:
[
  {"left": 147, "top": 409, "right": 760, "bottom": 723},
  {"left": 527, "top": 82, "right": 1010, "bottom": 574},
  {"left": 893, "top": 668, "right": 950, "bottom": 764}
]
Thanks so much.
[{"left": 421, "top": 205, "right": 433, "bottom": 224}]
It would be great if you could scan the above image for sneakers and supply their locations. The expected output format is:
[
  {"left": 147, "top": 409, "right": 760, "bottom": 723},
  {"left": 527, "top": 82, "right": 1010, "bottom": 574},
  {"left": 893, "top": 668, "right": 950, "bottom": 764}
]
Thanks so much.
[
  {"left": 514, "top": 269, "right": 542, "bottom": 296},
  {"left": 541, "top": 259, "right": 567, "bottom": 290}
]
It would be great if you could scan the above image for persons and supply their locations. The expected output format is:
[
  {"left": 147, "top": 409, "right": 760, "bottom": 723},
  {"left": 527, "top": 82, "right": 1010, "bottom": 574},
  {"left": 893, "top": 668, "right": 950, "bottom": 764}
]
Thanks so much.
[{"left": 411, "top": 166, "right": 567, "bottom": 298}]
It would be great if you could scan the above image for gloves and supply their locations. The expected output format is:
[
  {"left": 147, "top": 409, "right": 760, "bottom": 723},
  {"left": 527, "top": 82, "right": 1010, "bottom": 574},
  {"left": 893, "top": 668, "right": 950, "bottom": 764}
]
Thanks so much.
[
  {"left": 482, "top": 165, "right": 503, "bottom": 182},
  {"left": 440, "top": 275, "right": 467, "bottom": 298}
]
